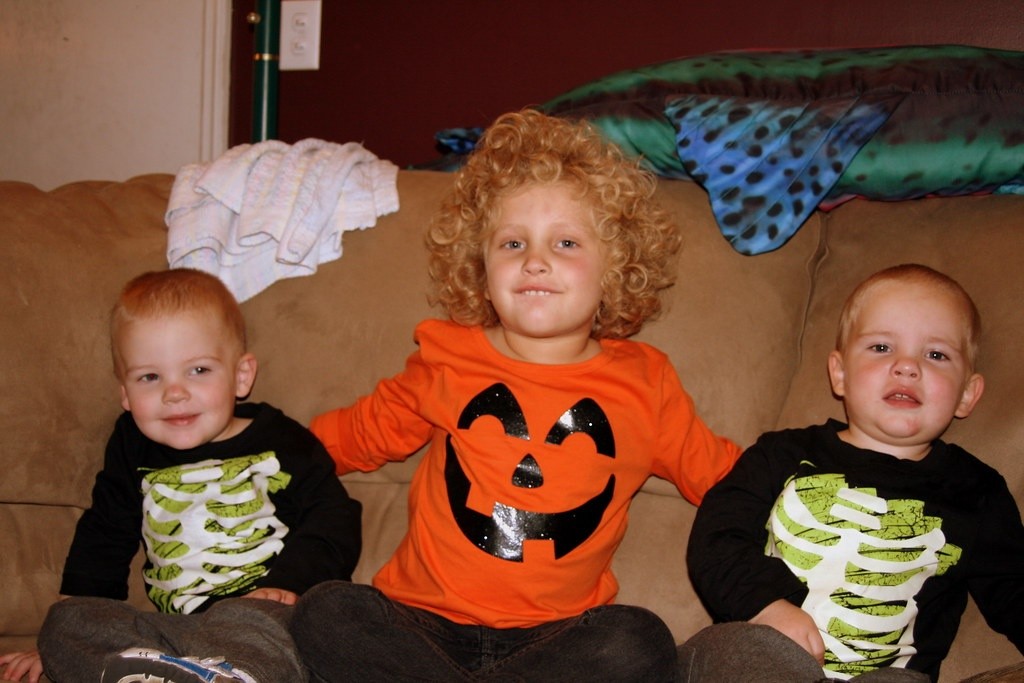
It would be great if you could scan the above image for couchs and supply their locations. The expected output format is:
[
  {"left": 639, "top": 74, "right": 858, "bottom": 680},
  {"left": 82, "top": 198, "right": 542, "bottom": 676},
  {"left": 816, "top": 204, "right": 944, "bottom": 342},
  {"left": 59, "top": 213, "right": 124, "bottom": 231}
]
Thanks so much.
[{"left": 0, "top": 168, "right": 1024, "bottom": 683}]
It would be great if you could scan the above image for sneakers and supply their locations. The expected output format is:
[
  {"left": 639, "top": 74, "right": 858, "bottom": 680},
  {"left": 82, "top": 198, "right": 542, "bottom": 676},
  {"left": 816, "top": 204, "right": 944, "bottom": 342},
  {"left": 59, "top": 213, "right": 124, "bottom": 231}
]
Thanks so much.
[{"left": 100, "top": 643, "right": 257, "bottom": 683}]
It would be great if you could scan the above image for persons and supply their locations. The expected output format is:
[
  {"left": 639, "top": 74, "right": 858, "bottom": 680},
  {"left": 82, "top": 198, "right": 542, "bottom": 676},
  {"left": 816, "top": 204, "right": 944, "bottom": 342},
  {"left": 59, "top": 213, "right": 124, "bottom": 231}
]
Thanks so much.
[
  {"left": 675, "top": 263, "right": 1023, "bottom": 683},
  {"left": 0, "top": 267, "right": 363, "bottom": 683},
  {"left": 291, "top": 108, "right": 744, "bottom": 683}
]
